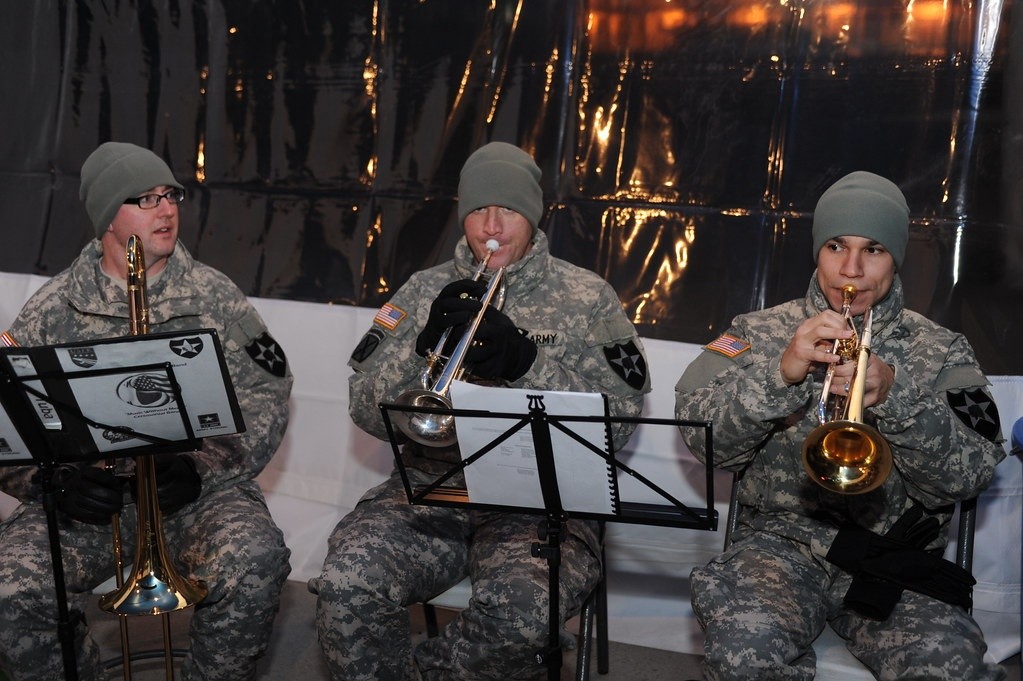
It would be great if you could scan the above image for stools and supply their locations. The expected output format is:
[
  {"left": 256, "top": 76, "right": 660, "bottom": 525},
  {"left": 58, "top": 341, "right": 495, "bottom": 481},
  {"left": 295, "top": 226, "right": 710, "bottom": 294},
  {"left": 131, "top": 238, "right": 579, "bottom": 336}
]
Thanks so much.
[{"left": 89, "top": 562, "right": 187, "bottom": 681}]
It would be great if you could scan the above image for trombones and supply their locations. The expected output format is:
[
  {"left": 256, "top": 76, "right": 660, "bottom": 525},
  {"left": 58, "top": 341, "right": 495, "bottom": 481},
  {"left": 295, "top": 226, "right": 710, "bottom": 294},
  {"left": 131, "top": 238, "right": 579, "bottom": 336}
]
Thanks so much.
[{"left": 97, "top": 234, "right": 210, "bottom": 681}]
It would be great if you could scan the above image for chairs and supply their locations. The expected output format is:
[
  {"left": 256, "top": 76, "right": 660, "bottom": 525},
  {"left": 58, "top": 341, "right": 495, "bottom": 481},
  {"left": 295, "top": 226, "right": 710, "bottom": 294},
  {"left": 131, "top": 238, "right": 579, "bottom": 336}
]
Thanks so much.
[
  {"left": 423, "top": 520, "right": 609, "bottom": 681},
  {"left": 724, "top": 473, "right": 977, "bottom": 618}
]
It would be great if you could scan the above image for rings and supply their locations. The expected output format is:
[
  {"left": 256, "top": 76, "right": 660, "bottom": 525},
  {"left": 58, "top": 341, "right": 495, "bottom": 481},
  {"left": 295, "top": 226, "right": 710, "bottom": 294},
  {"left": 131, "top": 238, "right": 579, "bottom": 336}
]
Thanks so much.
[{"left": 845, "top": 384, "right": 849, "bottom": 392}]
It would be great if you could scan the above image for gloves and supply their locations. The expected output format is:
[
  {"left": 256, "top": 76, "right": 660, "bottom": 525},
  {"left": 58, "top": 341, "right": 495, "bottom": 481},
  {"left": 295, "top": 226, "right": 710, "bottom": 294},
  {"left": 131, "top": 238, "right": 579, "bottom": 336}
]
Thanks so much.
[
  {"left": 415, "top": 278, "right": 537, "bottom": 381},
  {"left": 54, "top": 467, "right": 126, "bottom": 525},
  {"left": 825, "top": 503, "right": 976, "bottom": 621},
  {"left": 150, "top": 453, "right": 202, "bottom": 516}
]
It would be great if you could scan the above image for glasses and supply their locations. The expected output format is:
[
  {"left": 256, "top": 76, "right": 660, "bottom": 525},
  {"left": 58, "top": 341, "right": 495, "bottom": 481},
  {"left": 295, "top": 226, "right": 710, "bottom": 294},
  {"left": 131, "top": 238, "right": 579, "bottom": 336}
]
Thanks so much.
[{"left": 124, "top": 188, "right": 185, "bottom": 209}]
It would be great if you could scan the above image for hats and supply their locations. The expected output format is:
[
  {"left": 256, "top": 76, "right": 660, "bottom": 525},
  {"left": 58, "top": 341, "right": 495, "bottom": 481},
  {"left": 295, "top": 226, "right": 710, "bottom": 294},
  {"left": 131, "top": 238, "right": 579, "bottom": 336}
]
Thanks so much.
[
  {"left": 456, "top": 141, "right": 544, "bottom": 238},
  {"left": 79, "top": 141, "right": 184, "bottom": 241},
  {"left": 811, "top": 170, "right": 911, "bottom": 271}
]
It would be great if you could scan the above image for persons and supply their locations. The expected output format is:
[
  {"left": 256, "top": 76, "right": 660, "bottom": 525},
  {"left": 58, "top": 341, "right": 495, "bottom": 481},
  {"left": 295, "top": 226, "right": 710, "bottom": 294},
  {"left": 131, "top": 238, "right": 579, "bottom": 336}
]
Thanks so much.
[
  {"left": 674, "top": 171, "right": 1005, "bottom": 681},
  {"left": 309, "top": 142, "right": 653, "bottom": 681},
  {"left": 0, "top": 141, "right": 294, "bottom": 681}
]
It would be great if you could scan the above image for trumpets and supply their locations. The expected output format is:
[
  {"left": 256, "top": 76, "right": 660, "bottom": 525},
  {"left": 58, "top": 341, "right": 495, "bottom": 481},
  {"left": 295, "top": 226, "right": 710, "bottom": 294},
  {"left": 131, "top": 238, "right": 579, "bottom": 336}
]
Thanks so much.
[
  {"left": 800, "top": 283, "right": 893, "bottom": 495},
  {"left": 391, "top": 239, "right": 509, "bottom": 449}
]
